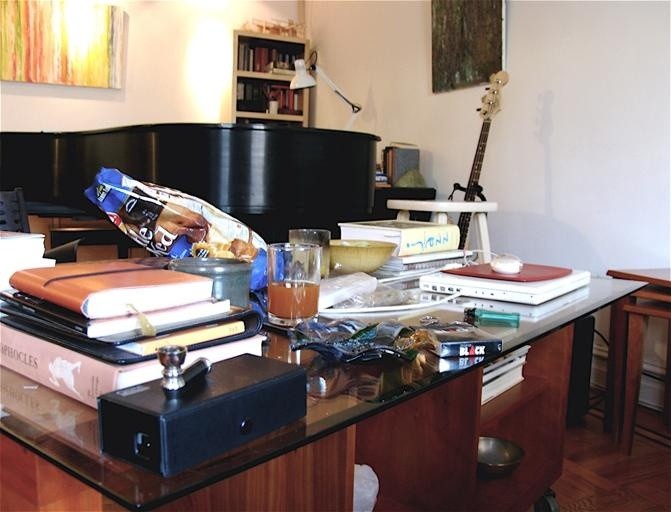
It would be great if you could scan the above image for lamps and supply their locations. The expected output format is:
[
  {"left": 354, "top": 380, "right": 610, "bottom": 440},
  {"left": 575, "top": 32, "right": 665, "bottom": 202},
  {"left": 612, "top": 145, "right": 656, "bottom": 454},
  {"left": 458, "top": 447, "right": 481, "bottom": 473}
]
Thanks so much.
[{"left": 289, "top": 59, "right": 361, "bottom": 114}]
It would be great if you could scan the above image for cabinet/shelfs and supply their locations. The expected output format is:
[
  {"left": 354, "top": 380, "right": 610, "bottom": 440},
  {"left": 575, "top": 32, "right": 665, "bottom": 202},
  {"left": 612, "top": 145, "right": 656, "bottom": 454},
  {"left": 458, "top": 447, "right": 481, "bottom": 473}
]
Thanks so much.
[{"left": 230, "top": 29, "right": 311, "bottom": 128}]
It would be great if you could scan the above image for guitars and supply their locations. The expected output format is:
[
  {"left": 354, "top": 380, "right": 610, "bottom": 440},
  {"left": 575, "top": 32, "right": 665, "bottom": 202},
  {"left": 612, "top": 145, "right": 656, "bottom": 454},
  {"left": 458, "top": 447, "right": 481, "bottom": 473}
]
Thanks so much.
[{"left": 457, "top": 70, "right": 510, "bottom": 249}]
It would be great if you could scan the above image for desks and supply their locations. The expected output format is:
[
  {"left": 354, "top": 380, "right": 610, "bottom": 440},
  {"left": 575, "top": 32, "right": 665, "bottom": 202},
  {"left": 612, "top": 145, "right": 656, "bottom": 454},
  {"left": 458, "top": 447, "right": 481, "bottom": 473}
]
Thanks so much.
[
  {"left": 0, "top": 260, "right": 649, "bottom": 512},
  {"left": 603, "top": 268, "right": 671, "bottom": 455}
]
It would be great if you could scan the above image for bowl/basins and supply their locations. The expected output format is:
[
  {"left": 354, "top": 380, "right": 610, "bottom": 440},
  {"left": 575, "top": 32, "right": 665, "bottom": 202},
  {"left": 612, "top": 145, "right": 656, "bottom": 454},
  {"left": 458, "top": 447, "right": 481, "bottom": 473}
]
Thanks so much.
[
  {"left": 329, "top": 239, "right": 399, "bottom": 274},
  {"left": 478, "top": 436, "right": 525, "bottom": 480}
]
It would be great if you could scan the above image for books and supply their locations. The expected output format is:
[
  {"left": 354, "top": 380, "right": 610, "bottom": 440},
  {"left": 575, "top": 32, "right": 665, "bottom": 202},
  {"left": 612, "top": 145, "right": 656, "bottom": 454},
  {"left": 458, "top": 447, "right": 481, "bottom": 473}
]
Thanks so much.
[
  {"left": 236, "top": 42, "right": 303, "bottom": 116},
  {"left": 376, "top": 141, "right": 420, "bottom": 189},
  {"left": 411, "top": 320, "right": 503, "bottom": 359},
  {"left": 422, "top": 353, "right": 486, "bottom": 372},
  {"left": 479, "top": 344, "right": 533, "bottom": 405},
  {"left": 338, "top": 220, "right": 462, "bottom": 258},
  {"left": 373, "top": 250, "right": 474, "bottom": 295},
  {"left": 1, "top": 230, "right": 57, "bottom": 290}
]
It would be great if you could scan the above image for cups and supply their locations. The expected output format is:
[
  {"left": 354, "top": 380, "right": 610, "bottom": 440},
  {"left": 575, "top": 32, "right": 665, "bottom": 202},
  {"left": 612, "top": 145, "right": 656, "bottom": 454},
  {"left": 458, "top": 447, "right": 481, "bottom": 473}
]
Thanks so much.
[
  {"left": 266, "top": 242, "right": 323, "bottom": 328},
  {"left": 289, "top": 228, "right": 332, "bottom": 278}
]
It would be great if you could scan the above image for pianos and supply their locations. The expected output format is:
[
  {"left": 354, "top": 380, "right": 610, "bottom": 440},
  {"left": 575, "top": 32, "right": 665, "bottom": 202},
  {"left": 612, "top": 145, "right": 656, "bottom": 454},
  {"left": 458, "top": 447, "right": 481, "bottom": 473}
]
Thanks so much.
[{"left": 1, "top": 123, "right": 382, "bottom": 259}]
